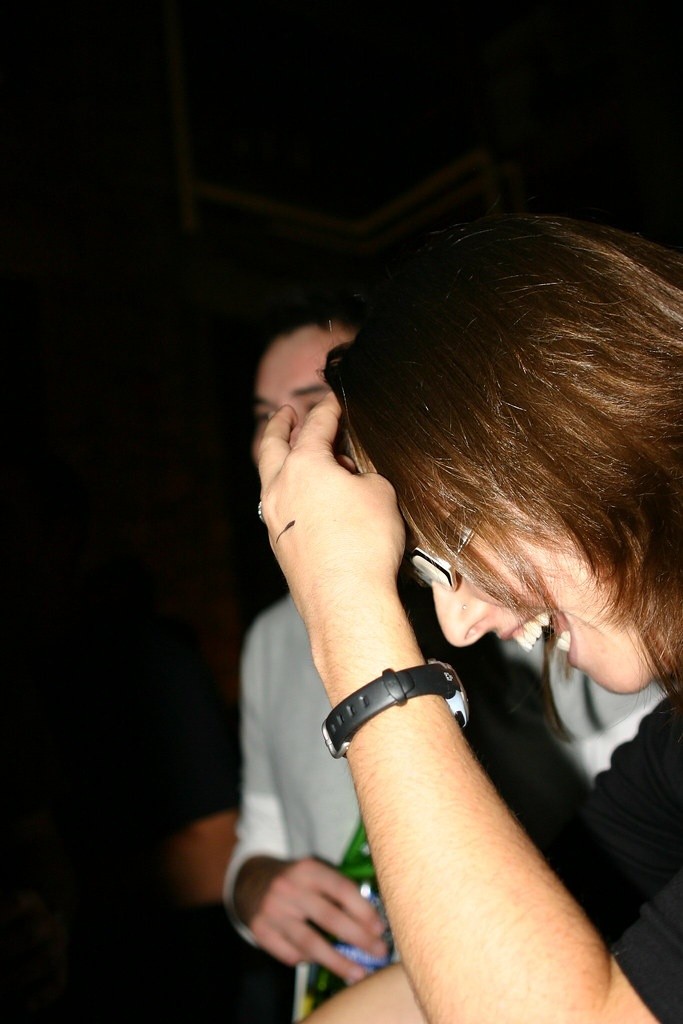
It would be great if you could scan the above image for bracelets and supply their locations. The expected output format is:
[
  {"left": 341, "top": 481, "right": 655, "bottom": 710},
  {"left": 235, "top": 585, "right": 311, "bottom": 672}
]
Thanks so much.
[{"left": 321, "top": 658, "right": 469, "bottom": 762}]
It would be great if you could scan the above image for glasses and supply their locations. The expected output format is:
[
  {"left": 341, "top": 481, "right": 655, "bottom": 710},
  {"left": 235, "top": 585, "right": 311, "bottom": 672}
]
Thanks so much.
[{"left": 410, "top": 473, "right": 502, "bottom": 595}]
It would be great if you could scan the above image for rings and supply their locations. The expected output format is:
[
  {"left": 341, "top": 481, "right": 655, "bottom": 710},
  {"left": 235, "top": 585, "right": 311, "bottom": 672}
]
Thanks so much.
[{"left": 258, "top": 501, "right": 265, "bottom": 523}]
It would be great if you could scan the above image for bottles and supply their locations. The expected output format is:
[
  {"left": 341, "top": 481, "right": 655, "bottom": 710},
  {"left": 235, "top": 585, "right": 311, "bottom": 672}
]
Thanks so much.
[{"left": 300, "top": 808, "right": 394, "bottom": 1017}]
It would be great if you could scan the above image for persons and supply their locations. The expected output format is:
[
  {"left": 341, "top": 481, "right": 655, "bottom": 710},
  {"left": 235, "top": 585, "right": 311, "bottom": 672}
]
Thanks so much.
[
  {"left": 221, "top": 297, "right": 666, "bottom": 1024},
  {"left": 261, "top": 216, "right": 683, "bottom": 1024}
]
